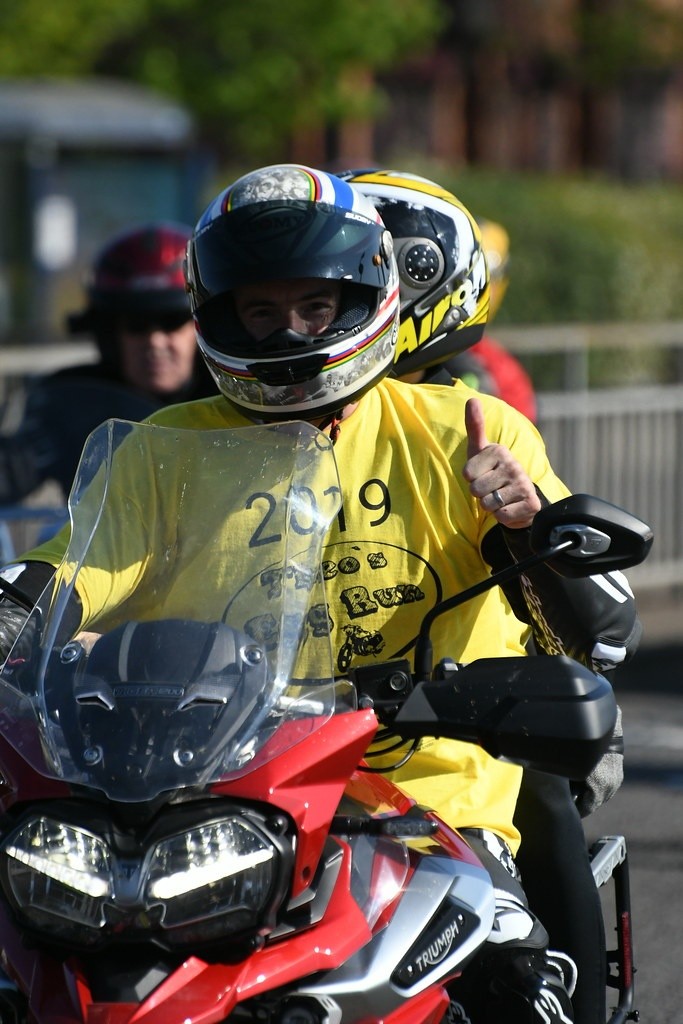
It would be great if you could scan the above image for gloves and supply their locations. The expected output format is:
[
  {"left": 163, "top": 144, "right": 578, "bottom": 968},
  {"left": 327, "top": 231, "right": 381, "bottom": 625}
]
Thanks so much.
[{"left": 0, "top": 602, "right": 38, "bottom": 681}]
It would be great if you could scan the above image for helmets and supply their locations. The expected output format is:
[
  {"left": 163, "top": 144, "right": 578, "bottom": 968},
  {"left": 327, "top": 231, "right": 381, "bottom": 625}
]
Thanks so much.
[
  {"left": 92, "top": 230, "right": 195, "bottom": 312},
  {"left": 335, "top": 172, "right": 491, "bottom": 380},
  {"left": 185, "top": 164, "right": 400, "bottom": 424}
]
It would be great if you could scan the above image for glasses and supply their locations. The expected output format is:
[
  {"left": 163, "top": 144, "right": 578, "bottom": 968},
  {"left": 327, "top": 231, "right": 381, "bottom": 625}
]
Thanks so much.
[{"left": 105, "top": 299, "right": 192, "bottom": 336}]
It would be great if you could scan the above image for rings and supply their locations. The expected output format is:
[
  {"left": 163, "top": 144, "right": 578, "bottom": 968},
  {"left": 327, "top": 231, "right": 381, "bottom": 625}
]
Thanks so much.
[{"left": 493, "top": 489, "right": 505, "bottom": 507}]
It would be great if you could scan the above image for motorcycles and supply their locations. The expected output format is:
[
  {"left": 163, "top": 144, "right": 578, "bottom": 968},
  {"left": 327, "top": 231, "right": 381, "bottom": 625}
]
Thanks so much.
[{"left": 1, "top": 420, "right": 654, "bottom": 1022}]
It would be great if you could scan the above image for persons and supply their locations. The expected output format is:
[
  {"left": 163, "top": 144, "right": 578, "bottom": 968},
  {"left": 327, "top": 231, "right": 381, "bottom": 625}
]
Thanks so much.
[
  {"left": 332, "top": 169, "right": 625, "bottom": 817},
  {"left": 466, "top": 216, "right": 537, "bottom": 428},
  {"left": 1, "top": 227, "right": 219, "bottom": 518},
  {"left": 0, "top": 162, "right": 637, "bottom": 1024}
]
